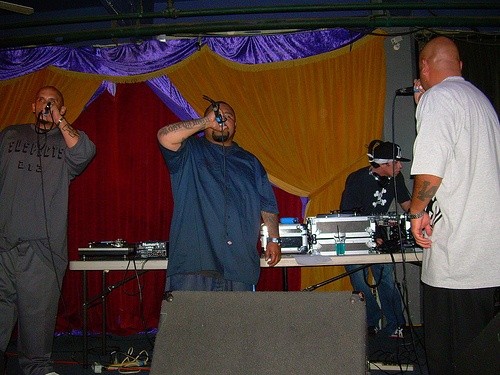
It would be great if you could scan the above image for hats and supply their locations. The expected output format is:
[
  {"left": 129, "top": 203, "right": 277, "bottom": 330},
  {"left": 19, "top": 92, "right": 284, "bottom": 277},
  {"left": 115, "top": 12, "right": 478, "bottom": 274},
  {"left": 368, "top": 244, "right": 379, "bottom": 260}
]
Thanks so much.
[{"left": 373, "top": 142, "right": 411, "bottom": 164}]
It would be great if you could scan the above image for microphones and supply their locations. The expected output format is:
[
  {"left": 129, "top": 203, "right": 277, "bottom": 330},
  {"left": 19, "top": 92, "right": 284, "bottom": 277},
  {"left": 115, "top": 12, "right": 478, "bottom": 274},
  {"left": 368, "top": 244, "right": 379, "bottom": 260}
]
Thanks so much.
[
  {"left": 395, "top": 86, "right": 414, "bottom": 96},
  {"left": 212, "top": 102, "right": 223, "bottom": 126},
  {"left": 46, "top": 100, "right": 51, "bottom": 112}
]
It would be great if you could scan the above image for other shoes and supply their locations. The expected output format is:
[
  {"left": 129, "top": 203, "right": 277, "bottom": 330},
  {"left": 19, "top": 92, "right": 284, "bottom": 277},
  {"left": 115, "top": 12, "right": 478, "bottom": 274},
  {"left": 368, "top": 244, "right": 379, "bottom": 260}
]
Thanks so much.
[
  {"left": 369, "top": 323, "right": 379, "bottom": 335},
  {"left": 388, "top": 326, "right": 404, "bottom": 338}
]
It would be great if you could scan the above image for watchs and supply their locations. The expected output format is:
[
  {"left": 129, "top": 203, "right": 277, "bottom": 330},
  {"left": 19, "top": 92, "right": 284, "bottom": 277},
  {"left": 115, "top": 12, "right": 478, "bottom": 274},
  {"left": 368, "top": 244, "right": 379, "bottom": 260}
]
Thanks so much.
[{"left": 267, "top": 237, "right": 282, "bottom": 246}]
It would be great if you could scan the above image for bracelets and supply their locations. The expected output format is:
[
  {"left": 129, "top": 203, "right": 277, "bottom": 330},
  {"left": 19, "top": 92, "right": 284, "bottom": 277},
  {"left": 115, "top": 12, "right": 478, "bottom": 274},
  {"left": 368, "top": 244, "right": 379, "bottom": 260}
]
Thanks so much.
[
  {"left": 407, "top": 210, "right": 424, "bottom": 219},
  {"left": 56, "top": 117, "right": 64, "bottom": 127}
]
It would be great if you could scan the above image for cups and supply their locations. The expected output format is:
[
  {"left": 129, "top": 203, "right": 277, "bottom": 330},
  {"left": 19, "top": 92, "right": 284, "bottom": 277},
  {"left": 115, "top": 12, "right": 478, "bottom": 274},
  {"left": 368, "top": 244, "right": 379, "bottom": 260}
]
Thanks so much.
[{"left": 334, "top": 232, "right": 346, "bottom": 256}]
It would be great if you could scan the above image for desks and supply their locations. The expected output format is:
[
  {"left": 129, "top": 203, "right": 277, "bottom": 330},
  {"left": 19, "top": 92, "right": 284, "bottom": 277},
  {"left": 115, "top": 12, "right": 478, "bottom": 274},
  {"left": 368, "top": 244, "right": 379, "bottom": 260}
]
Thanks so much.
[{"left": 68, "top": 247, "right": 424, "bottom": 370}]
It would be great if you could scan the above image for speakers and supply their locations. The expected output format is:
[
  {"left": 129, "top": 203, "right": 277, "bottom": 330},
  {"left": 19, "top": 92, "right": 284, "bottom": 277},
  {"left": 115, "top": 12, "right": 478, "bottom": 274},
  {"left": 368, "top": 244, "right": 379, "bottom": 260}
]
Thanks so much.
[{"left": 149, "top": 290, "right": 368, "bottom": 375}]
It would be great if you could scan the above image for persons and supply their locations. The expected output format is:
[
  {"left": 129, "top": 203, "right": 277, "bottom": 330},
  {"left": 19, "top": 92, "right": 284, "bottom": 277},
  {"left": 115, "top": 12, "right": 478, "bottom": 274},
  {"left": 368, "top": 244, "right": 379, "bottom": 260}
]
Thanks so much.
[
  {"left": 409, "top": 36, "right": 500, "bottom": 375},
  {"left": 157, "top": 100, "right": 282, "bottom": 293},
  {"left": 340, "top": 141, "right": 413, "bottom": 346},
  {"left": 0, "top": 86, "right": 96, "bottom": 375}
]
